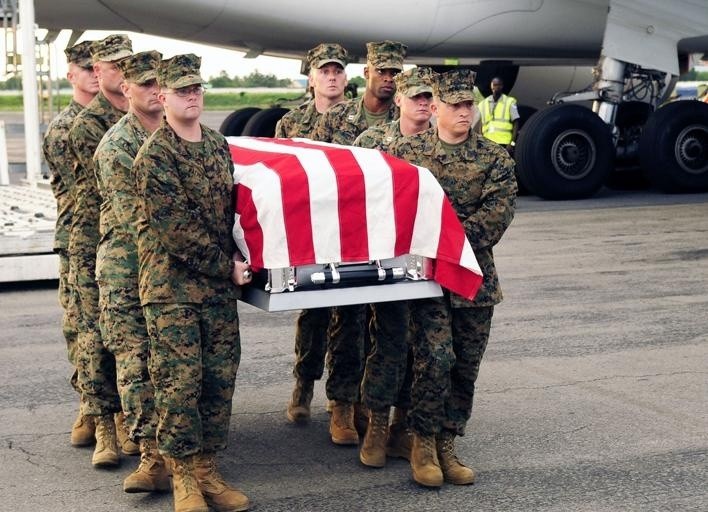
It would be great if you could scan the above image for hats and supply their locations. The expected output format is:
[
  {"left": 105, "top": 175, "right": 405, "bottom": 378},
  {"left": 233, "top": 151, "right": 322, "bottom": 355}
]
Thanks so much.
[
  {"left": 87, "top": 33, "right": 134, "bottom": 63},
  {"left": 365, "top": 40, "right": 408, "bottom": 71},
  {"left": 63, "top": 40, "right": 97, "bottom": 69},
  {"left": 115, "top": 50, "right": 163, "bottom": 86},
  {"left": 428, "top": 69, "right": 477, "bottom": 105},
  {"left": 392, "top": 67, "right": 440, "bottom": 99},
  {"left": 306, "top": 43, "right": 349, "bottom": 70},
  {"left": 154, "top": 53, "right": 208, "bottom": 90}
]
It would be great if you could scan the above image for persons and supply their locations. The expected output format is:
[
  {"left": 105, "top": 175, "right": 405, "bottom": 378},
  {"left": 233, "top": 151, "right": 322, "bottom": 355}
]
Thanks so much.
[
  {"left": 92, "top": 51, "right": 172, "bottom": 494},
  {"left": 133, "top": 54, "right": 253, "bottom": 512},
  {"left": 311, "top": 40, "right": 408, "bottom": 448},
  {"left": 41, "top": 39, "right": 99, "bottom": 448},
  {"left": 349, "top": 66, "right": 438, "bottom": 469},
  {"left": 67, "top": 35, "right": 139, "bottom": 469},
  {"left": 275, "top": 43, "right": 338, "bottom": 424},
  {"left": 477, "top": 78, "right": 520, "bottom": 155},
  {"left": 390, "top": 70, "right": 517, "bottom": 488}
]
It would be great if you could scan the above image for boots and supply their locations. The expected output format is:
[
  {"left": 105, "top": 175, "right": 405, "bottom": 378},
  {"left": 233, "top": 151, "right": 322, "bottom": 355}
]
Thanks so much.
[
  {"left": 91, "top": 413, "right": 120, "bottom": 468},
  {"left": 193, "top": 451, "right": 250, "bottom": 512},
  {"left": 386, "top": 407, "right": 414, "bottom": 462},
  {"left": 113, "top": 410, "right": 141, "bottom": 456},
  {"left": 358, "top": 405, "right": 391, "bottom": 469},
  {"left": 326, "top": 398, "right": 371, "bottom": 446},
  {"left": 70, "top": 398, "right": 96, "bottom": 447},
  {"left": 122, "top": 436, "right": 174, "bottom": 494},
  {"left": 409, "top": 430, "right": 445, "bottom": 487},
  {"left": 435, "top": 430, "right": 474, "bottom": 486},
  {"left": 287, "top": 378, "right": 315, "bottom": 425},
  {"left": 161, "top": 453, "right": 209, "bottom": 512}
]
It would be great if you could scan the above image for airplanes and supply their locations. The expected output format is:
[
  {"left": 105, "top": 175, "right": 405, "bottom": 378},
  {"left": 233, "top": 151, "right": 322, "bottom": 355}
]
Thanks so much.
[{"left": 0, "top": 1, "right": 707, "bottom": 204}]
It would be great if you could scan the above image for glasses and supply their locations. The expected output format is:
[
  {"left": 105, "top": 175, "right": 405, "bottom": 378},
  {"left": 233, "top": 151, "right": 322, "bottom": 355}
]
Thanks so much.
[{"left": 161, "top": 85, "right": 208, "bottom": 97}]
